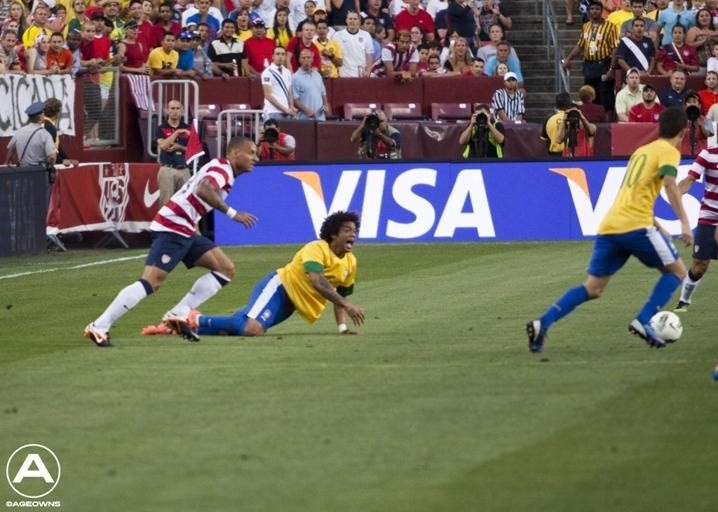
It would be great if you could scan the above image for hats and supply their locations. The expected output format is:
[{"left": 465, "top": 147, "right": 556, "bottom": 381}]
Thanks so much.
[
  {"left": 625, "top": 66, "right": 640, "bottom": 78},
  {"left": 643, "top": 84, "right": 657, "bottom": 93},
  {"left": 122, "top": 20, "right": 140, "bottom": 30},
  {"left": 684, "top": 92, "right": 702, "bottom": 103},
  {"left": 24, "top": 101, "right": 48, "bottom": 117},
  {"left": 312, "top": 6, "right": 326, "bottom": 15},
  {"left": 503, "top": 71, "right": 518, "bottom": 82},
  {"left": 250, "top": 17, "right": 267, "bottom": 27},
  {"left": 179, "top": 30, "right": 202, "bottom": 41},
  {"left": 90, "top": 11, "right": 108, "bottom": 21},
  {"left": 101, "top": 1, "right": 120, "bottom": 8}
]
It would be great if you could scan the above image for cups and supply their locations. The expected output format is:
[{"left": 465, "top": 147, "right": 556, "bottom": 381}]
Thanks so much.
[
  {"left": 358, "top": 64, "right": 366, "bottom": 79},
  {"left": 323, "top": 66, "right": 331, "bottom": 78}
]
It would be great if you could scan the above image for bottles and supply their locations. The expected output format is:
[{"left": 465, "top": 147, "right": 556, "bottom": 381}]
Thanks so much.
[
  {"left": 263, "top": 58, "right": 268, "bottom": 69},
  {"left": 232, "top": 58, "right": 239, "bottom": 77}
]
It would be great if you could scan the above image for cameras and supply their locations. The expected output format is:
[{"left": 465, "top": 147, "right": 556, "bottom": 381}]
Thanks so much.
[{"left": 476, "top": 113, "right": 487, "bottom": 123}]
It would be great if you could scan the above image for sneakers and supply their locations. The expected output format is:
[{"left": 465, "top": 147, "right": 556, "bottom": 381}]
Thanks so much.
[
  {"left": 673, "top": 299, "right": 692, "bottom": 312},
  {"left": 628, "top": 317, "right": 667, "bottom": 349},
  {"left": 82, "top": 321, "right": 112, "bottom": 347},
  {"left": 139, "top": 320, "right": 177, "bottom": 336},
  {"left": 526, "top": 318, "right": 548, "bottom": 353},
  {"left": 160, "top": 309, "right": 202, "bottom": 342}
]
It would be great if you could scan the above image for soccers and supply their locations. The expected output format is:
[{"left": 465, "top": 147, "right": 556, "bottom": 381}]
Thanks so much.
[{"left": 649, "top": 310, "right": 683, "bottom": 343}]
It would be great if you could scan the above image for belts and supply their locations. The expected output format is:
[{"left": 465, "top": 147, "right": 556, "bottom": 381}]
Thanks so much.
[{"left": 167, "top": 164, "right": 189, "bottom": 169}]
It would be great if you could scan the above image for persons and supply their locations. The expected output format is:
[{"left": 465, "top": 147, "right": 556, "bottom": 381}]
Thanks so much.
[
  {"left": 523, "top": 105, "right": 693, "bottom": 355},
  {"left": 153, "top": 99, "right": 190, "bottom": 211},
  {"left": 140, "top": 210, "right": 365, "bottom": 338},
  {"left": 84, "top": 135, "right": 259, "bottom": 347},
  {"left": 673, "top": 146, "right": 718, "bottom": 313}
]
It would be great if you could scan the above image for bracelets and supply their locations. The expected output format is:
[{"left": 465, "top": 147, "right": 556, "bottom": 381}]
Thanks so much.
[
  {"left": 337, "top": 324, "right": 348, "bottom": 334},
  {"left": 226, "top": 206, "right": 237, "bottom": 218}
]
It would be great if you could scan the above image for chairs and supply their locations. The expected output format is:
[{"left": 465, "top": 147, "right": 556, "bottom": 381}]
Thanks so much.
[{"left": 188, "top": 100, "right": 497, "bottom": 140}]
